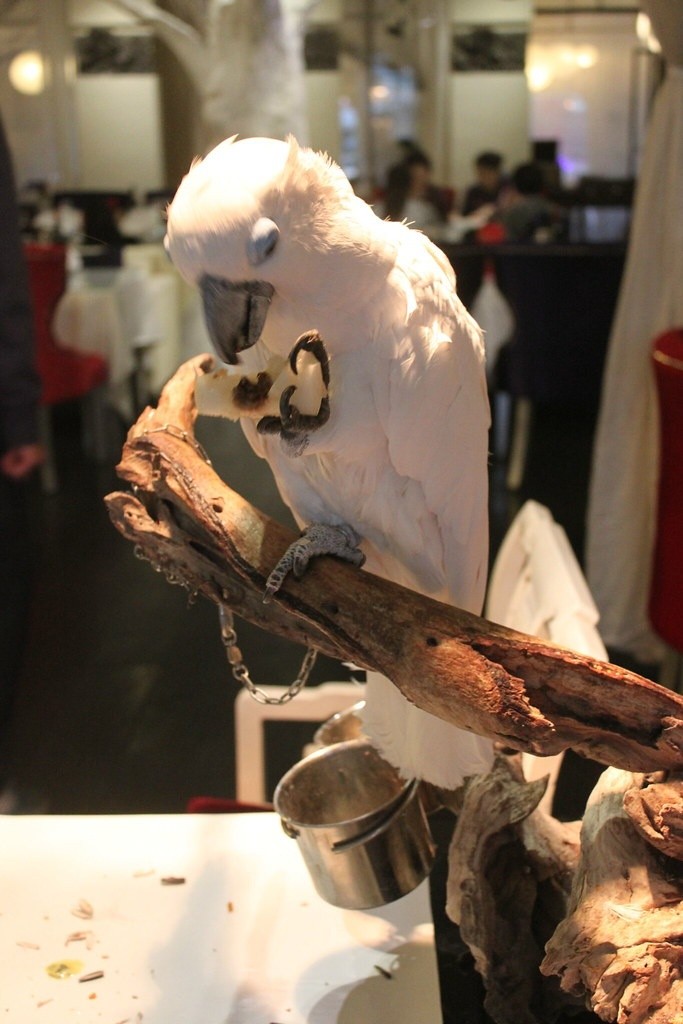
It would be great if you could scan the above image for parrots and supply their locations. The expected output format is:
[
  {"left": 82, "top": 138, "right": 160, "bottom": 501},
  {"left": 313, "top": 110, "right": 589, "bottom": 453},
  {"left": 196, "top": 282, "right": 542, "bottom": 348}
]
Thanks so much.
[{"left": 164, "top": 138, "right": 494, "bottom": 789}]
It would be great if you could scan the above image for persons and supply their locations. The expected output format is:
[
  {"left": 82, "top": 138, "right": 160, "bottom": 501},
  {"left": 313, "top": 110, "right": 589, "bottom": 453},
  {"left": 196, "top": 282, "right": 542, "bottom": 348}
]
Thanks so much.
[
  {"left": 0, "top": 107, "right": 49, "bottom": 812},
  {"left": 383, "top": 139, "right": 571, "bottom": 242}
]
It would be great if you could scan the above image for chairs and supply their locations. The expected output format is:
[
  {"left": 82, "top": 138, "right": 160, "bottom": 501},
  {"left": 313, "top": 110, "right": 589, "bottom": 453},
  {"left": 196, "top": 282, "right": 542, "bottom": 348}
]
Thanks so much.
[{"left": 19, "top": 242, "right": 109, "bottom": 498}]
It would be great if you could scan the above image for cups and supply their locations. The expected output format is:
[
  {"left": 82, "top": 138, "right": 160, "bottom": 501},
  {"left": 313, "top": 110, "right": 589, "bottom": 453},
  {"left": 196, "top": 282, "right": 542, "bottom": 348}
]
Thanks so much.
[{"left": 272, "top": 738, "right": 438, "bottom": 911}]
